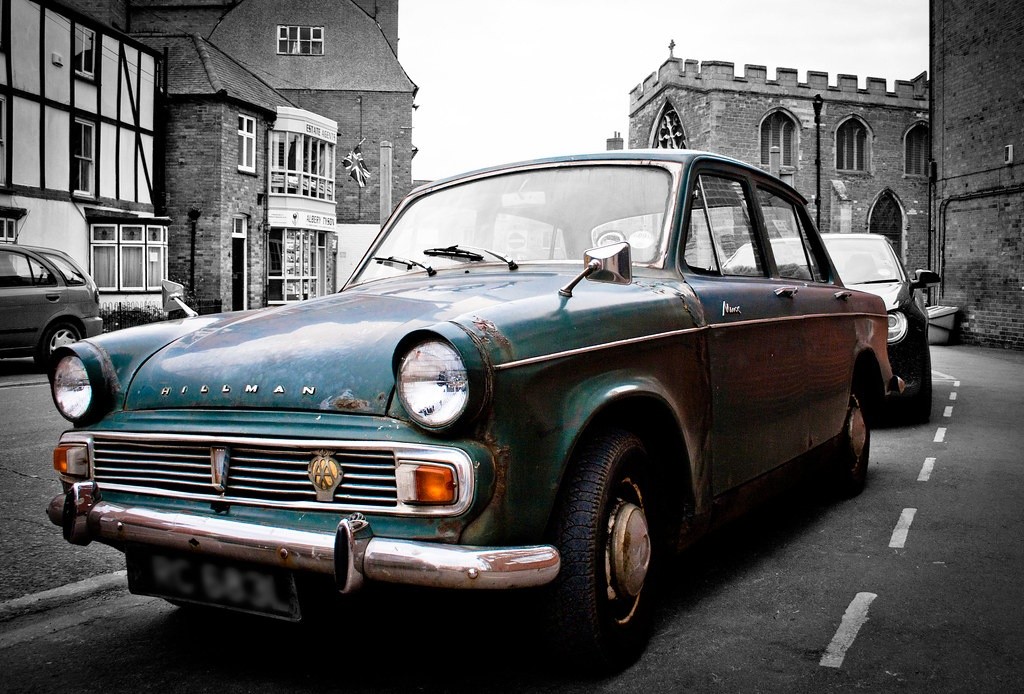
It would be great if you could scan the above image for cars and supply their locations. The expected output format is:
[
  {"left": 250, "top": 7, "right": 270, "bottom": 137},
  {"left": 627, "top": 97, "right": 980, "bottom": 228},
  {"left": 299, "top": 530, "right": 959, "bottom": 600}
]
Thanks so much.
[
  {"left": 719, "top": 232, "right": 942, "bottom": 424},
  {"left": 43, "top": 150, "right": 906, "bottom": 686},
  {"left": 0, "top": 242, "right": 104, "bottom": 372}
]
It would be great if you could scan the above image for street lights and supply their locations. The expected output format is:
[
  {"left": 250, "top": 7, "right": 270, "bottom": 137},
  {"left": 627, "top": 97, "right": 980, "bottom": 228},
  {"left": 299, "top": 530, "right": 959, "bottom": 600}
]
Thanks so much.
[{"left": 811, "top": 92, "right": 824, "bottom": 231}]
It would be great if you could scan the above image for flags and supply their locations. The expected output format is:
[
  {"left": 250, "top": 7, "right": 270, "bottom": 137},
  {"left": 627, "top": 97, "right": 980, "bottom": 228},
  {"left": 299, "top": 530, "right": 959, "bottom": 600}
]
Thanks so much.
[{"left": 342, "top": 146, "right": 372, "bottom": 188}]
